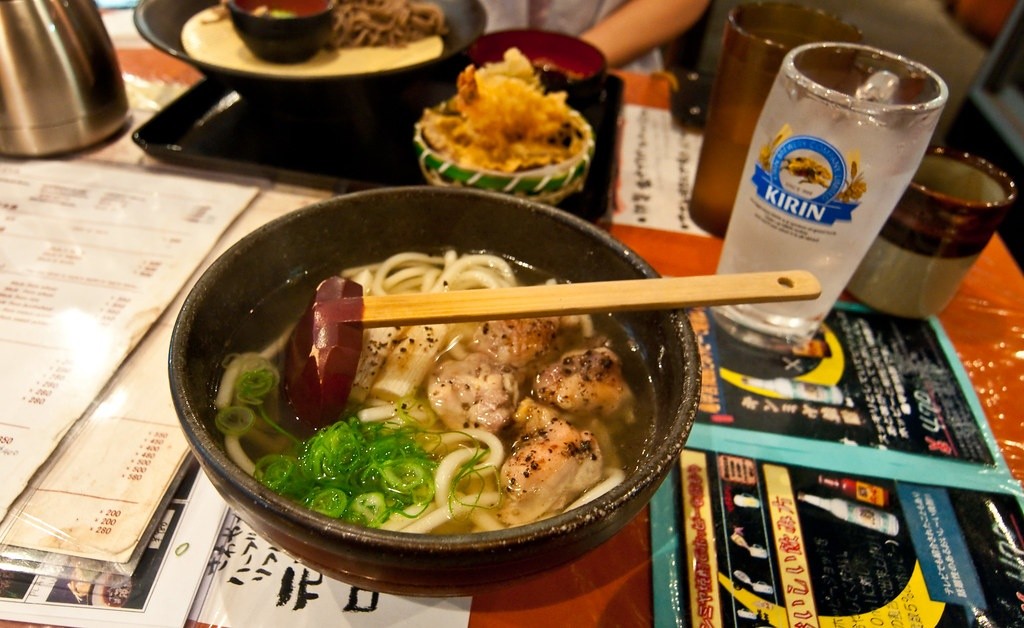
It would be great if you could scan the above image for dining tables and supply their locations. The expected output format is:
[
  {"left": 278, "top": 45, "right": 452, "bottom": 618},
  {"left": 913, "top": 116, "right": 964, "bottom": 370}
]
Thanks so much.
[{"left": 0, "top": 3, "right": 1024, "bottom": 628}]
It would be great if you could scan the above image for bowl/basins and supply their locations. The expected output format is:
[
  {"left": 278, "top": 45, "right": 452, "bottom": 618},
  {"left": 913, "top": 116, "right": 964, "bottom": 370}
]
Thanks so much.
[
  {"left": 133, "top": 1, "right": 488, "bottom": 93},
  {"left": 167, "top": 185, "right": 703, "bottom": 595},
  {"left": 469, "top": 28, "right": 607, "bottom": 106},
  {"left": 229, "top": 0, "right": 336, "bottom": 64},
  {"left": 415, "top": 98, "right": 595, "bottom": 203}
]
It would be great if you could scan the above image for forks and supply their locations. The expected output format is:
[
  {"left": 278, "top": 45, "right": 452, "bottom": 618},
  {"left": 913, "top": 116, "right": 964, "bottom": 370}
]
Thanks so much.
[{"left": 147, "top": 90, "right": 241, "bottom": 153}]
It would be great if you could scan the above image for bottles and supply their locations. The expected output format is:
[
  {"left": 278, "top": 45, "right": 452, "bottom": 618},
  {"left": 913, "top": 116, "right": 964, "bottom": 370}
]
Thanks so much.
[
  {"left": 818, "top": 475, "right": 889, "bottom": 508},
  {"left": 741, "top": 377, "right": 844, "bottom": 405},
  {"left": 798, "top": 493, "right": 900, "bottom": 536}
]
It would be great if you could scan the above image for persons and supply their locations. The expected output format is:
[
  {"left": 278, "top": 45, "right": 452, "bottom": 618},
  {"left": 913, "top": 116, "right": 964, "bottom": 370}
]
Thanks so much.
[{"left": 479, "top": 0, "right": 710, "bottom": 74}]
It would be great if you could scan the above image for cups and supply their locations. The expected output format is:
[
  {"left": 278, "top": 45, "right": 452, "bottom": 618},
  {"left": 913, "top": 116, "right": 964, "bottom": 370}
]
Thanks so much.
[
  {"left": 690, "top": 0, "right": 865, "bottom": 241},
  {"left": 846, "top": 144, "right": 1016, "bottom": 320},
  {"left": 734, "top": 493, "right": 760, "bottom": 508},
  {"left": 710, "top": 42, "right": 950, "bottom": 355}
]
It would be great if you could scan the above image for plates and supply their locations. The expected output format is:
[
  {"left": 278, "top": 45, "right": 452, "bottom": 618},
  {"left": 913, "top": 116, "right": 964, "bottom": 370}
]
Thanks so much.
[{"left": 181, "top": 6, "right": 443, "bottom": 77}]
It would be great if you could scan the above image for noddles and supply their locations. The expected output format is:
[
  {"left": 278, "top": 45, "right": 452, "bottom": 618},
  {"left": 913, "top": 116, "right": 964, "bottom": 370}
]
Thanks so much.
[{"left": 212, "top": 248, "right": 656, "bottom": 539}]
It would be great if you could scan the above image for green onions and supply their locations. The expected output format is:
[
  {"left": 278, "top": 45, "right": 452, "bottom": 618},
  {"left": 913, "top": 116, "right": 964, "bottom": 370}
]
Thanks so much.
[{"left": 214, "top": 349, "right": 503, "bottom": 528}]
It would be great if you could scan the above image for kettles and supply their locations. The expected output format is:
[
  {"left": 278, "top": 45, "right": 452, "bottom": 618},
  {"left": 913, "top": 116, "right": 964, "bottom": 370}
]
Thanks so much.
[{"left": 0, "top": 0, "right": 129, "bottom": 157}]
